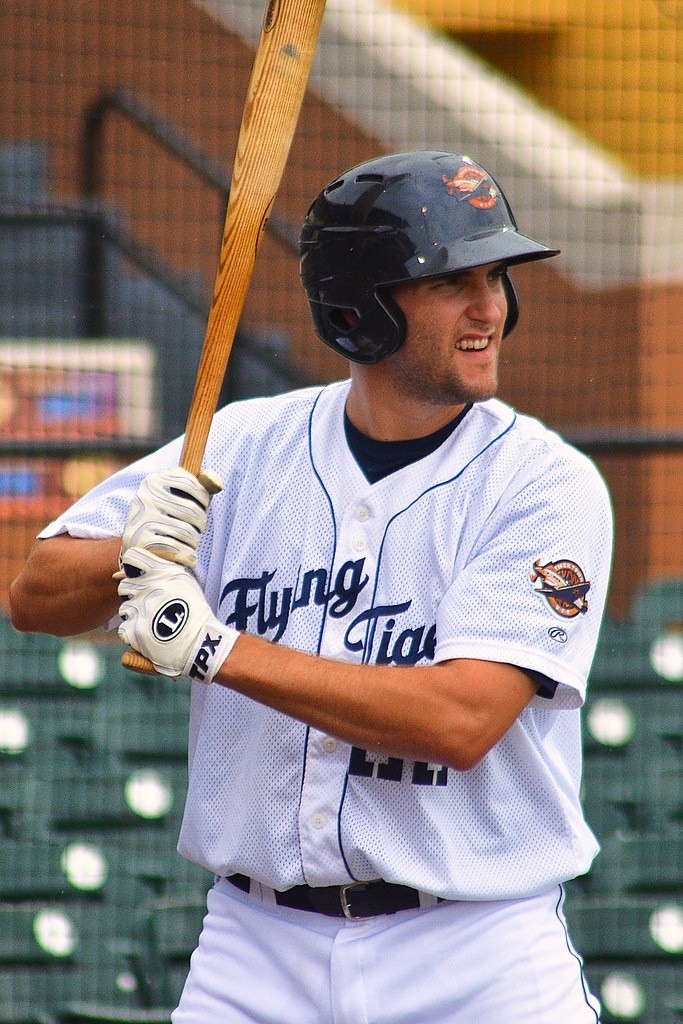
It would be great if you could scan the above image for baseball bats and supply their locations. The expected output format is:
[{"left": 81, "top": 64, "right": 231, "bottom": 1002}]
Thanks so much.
[{"left": 113, "top": 1, "right": 332, "bottom": 680}]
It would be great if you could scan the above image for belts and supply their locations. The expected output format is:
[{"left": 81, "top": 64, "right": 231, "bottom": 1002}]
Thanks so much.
[{"left": 226, "top": 872, "right": 448, "bottom": 922}]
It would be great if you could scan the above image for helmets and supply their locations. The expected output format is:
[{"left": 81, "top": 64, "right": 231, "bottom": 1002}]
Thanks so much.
[{"left": 299, "top": 150, "right": 561, "bottom": 364}]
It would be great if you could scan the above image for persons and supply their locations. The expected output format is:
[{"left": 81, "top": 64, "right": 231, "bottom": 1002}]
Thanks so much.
[{"left": 7, "top": 147, "right": 615, "bottom": 1024}]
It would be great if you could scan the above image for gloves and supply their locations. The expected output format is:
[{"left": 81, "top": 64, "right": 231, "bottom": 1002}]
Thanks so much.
[
  {"left": 112, "top": 547, "right": 240, "bottom": 685},
  {"left": 118, "top": 468, "right": 223, "bottom": 571}
]
